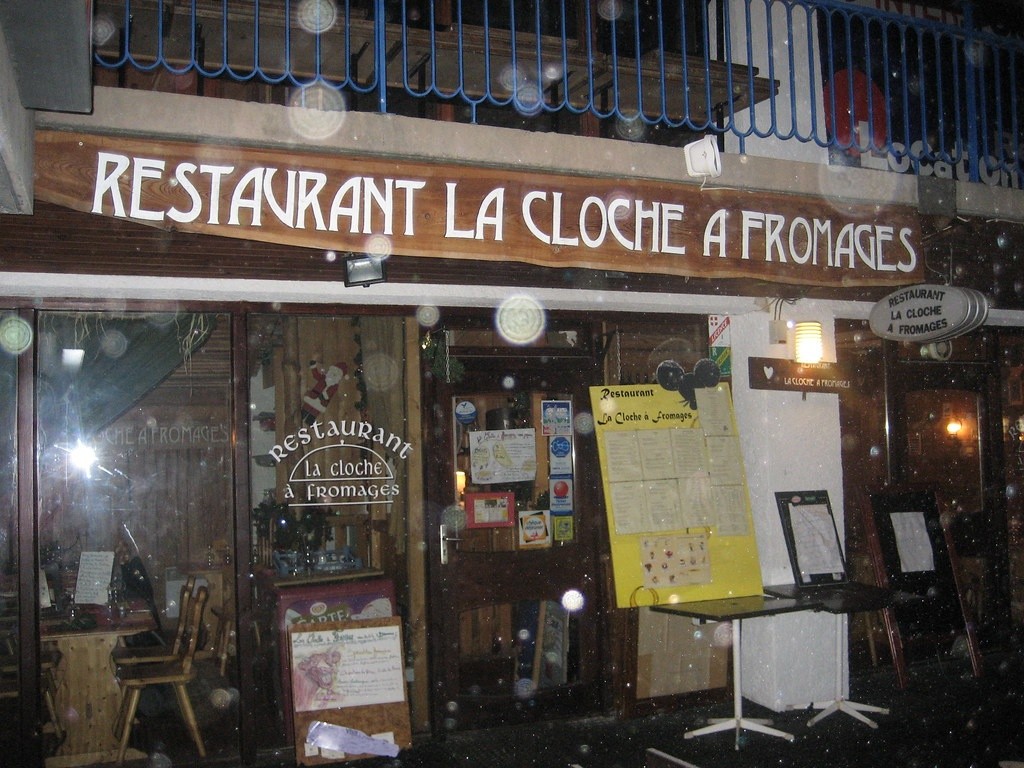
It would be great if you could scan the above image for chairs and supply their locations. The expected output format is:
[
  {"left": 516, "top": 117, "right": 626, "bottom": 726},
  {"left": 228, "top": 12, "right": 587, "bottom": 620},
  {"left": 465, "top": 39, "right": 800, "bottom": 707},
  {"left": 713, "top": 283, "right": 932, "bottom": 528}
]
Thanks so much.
[{"left": 0, "top": 556, "right": 263, "bottom": 768}]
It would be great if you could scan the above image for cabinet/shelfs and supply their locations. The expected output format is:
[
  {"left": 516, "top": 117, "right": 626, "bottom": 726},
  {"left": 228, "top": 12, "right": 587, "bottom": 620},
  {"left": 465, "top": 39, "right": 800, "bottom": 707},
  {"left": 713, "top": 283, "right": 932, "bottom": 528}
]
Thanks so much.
[{"left": 240, "top": 558, "right": 385, "bottom": 755}]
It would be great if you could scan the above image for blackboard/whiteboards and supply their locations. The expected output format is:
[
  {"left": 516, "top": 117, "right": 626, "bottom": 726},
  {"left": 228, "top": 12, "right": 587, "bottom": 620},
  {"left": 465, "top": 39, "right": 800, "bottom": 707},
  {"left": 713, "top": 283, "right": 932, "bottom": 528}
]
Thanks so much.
[{"left": 854, "top": 480, "right": 977, "bottom": 668}]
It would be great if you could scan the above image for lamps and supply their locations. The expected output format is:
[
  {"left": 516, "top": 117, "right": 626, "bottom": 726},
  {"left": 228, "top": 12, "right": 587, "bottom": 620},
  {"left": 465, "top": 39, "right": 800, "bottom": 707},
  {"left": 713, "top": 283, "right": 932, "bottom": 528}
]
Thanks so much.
[
  {"left": 794, "top": 322, "right": 824, "bottom": 366},
  {"left": 341, "top": 252, "right": 385, "bottom": 290},
  {"left": 683, "top": 135, "right": 721, "bottom": 179}
]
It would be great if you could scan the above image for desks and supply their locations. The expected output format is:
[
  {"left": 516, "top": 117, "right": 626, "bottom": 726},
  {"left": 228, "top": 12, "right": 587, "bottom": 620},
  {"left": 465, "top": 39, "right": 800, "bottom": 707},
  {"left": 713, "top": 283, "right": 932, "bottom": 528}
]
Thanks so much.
[
  {"left": 92, "top": 0, "right": 782, "bottom": 153},
  {"left": 655, "top": 580, "right": 892, "bottom": 749}
]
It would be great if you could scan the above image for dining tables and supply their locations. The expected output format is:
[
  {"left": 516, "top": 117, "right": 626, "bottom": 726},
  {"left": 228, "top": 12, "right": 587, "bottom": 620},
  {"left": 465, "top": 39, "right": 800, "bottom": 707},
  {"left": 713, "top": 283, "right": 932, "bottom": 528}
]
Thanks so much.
[{"left": 38, "top": 594, "right": 159, "bottom": 768}]
